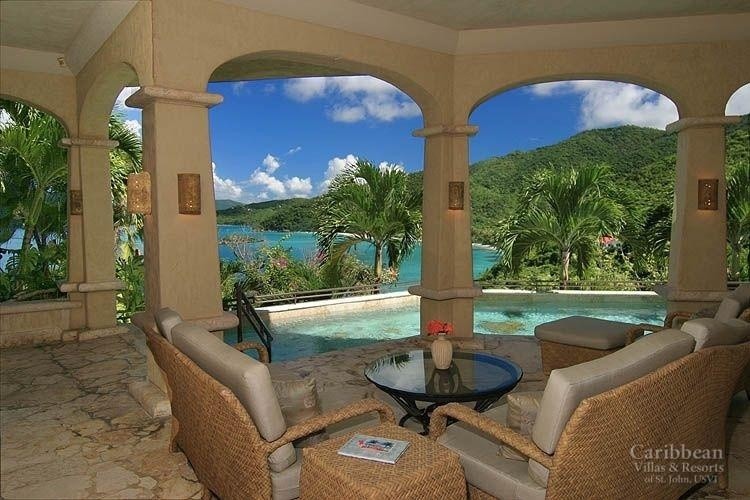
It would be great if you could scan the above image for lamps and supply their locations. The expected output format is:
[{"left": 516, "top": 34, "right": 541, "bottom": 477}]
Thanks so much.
[
  {"left": 127, "top": 172, "right": 153, "bottom": 215},
  {"left": 447, "top": 182, "right": 465, "bottom": 210},
  {"left": 70, "top": 190, "right": 82, "bottom": 214},
  {"left": 698, "top": 178, "right": 718, "bottom": 212},
  {"left": 179, "top": 173, "right": 200, "bottom": 214}
]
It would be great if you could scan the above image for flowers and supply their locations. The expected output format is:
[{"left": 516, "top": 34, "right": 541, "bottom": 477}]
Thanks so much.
[{"left": 425, "top": 318, "right": 453, "bottom": 336}]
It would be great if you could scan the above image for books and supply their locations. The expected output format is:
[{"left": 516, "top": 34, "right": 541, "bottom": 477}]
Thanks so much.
[{"left": 337, "top": 433, "right": 412, "bottom": 465}]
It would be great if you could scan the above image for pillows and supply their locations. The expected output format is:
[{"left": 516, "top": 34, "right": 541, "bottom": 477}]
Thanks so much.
[
  {"left": 713, "top": 282, "right": 750, "bottom": 319},
  {"left": 266, "top": 375, "right": 329, "bottom": 449},
  {"left": 495, "top": 389, "right": 545, "bottom": 460}
]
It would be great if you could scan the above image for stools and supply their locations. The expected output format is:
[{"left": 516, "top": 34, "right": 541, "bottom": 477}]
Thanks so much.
[{"left": 531, "top": 317, "right": 643, "bottom": 376}]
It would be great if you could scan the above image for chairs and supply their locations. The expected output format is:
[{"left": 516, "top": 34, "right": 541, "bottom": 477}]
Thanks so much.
[
  {"left": 427, "top": 315, "right": 750, "bottom": 500},
  {"left": 130, "top": 306, "right": 395, "bottom": 499},
  {"left": 625, "top": 282, "right": 750, "bottom": 346}
]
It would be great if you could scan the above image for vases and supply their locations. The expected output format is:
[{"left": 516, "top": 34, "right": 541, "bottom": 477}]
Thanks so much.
[{"left": 431, "top": 332, "right": 453, "bottom": 370}]
